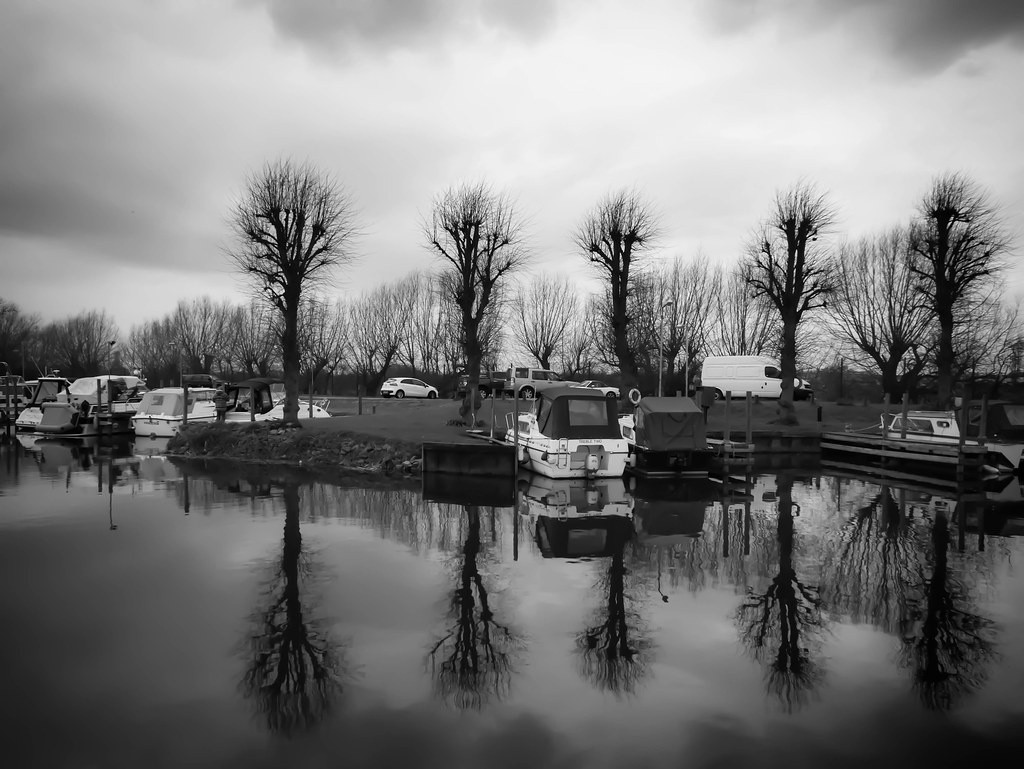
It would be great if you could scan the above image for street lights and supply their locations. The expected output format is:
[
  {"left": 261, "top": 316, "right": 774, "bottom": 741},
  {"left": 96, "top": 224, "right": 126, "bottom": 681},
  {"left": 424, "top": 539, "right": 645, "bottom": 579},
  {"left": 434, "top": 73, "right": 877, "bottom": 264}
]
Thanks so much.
[
  {"left": 658, "top": 300, "right": 673, "bottom": 397},
  {"left": 107, "top": 340, "right": 116, "bottom": 375}
]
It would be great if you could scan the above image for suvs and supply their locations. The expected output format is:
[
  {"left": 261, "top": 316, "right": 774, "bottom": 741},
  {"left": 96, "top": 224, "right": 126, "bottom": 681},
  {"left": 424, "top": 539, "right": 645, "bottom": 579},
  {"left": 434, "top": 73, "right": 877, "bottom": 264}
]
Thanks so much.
[{"left": 506, "top": 366, "right": 582, "bottom": 401}]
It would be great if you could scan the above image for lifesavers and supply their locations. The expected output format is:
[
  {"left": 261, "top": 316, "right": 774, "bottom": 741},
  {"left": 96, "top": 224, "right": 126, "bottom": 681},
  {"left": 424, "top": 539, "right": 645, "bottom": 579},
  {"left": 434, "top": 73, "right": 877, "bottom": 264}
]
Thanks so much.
[{"left": 629, "top": 388, "right": 642, "bottom": 405}]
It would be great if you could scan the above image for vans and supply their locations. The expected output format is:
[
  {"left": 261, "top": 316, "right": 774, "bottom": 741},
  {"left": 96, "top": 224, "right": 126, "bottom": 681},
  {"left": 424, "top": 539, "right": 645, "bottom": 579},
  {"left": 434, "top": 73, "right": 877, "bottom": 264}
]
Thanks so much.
[{"left": 701, "top": 354, "right": 815, "bottom": 402}]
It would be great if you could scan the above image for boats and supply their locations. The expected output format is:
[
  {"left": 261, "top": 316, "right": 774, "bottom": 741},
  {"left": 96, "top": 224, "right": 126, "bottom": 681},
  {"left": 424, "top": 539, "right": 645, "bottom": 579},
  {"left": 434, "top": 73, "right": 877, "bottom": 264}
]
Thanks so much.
[
  {"left": 882, "top": 410, "right": 1024, "bottom": 477},
  {"left": 504, "top": 386, "right": 629, "bottom": 480},
  {"left": 512, "top": 462, "right": 635, "bottom": 560},
  {"left": 616, "top": 395, "right": 712, "bottom": 479},
  {"left": 11, "top": 375, "right": 333, "bottom": 439}
]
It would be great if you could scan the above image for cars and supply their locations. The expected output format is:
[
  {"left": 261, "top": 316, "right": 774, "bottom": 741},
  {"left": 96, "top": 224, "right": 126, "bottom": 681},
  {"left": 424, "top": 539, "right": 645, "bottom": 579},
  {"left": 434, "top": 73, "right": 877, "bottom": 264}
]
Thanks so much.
[
  {"left": 379, "top": 377, "right": 439, "bottom": 399},
  {"left": 570, "top": 380, "right": 621, "bottom": 401}
]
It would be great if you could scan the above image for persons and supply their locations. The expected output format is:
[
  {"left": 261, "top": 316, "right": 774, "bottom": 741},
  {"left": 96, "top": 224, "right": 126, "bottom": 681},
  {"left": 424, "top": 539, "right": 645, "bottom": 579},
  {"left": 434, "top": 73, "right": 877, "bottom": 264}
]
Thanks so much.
[{"left": 212, "top": 386, "right": 229, "bottom": 422}]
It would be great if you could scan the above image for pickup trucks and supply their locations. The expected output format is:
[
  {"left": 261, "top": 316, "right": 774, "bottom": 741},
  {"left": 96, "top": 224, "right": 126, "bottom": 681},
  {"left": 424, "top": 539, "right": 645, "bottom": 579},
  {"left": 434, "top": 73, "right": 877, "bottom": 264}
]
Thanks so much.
[{"left": 458, "top": 370, "right": 513, "bottom": 400}]
